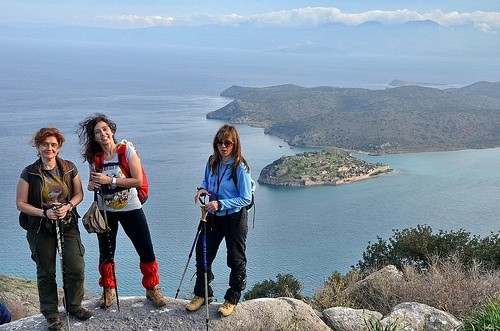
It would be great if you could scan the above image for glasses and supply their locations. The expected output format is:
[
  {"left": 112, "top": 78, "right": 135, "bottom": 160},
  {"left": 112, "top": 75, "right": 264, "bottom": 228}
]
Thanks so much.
[{"left": 215, "top": 139, "right": 234, "bottom": 147}]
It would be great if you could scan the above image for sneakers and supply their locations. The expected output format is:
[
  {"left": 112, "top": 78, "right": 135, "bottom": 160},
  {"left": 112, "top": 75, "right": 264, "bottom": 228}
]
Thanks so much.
[
  {"left": 46, "top": 315, "right": 62, "bottom": 331},
  {"left": 68, "top": 307, "right": 92, "bottom": 320},
  {"left": 185, "top": 296, "right": 214, "bottom": 312},
  {"left": 217, "top": 299, "right": 236, "bottom": 317},
  {"left": 146, "top": 284, "right": 166, "bottom": 307},
  {"left": 99, "top": 288, "right": 116, "bottom": 308}
]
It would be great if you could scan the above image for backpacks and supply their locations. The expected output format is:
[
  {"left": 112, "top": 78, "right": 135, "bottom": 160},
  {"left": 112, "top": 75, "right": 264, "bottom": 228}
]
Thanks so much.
[
  {"left": 93, "top": 144, "right": 148, "bottom": 206},
  {"left": 208, "top": 153, "right": 257, "bottom": 213}
]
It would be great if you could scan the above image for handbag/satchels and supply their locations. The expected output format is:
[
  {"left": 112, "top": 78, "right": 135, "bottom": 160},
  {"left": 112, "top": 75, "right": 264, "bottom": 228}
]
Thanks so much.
[{"left": 82, "top": 202, "right": 111, "bottom": 233}]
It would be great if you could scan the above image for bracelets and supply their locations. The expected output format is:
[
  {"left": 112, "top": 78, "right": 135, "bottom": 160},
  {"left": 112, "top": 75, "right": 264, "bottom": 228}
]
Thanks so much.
[
  {"left": 217, "top": 201, "right": 221, "bottom": 211},
  {"left": 43, "top": 208, "right": 50, "bottom": 219}
]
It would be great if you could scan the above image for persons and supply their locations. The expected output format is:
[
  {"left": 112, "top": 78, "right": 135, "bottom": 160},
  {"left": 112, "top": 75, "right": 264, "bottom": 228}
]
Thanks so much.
[
  {"left": 75, "top": 111, "right": 167, "bottom": 308},
  {"left": 15, "top": 127, "right": 93, "bottom": 331},
  {"left": 185, "top": 124, "right": 253, "bottom": 318}
]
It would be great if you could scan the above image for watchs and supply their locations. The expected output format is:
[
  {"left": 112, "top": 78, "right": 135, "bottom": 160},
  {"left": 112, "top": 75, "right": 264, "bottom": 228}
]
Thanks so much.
[
  {"left": 67, "top": 201, "right": 74, "bottom": 211},
  {"left": 108, "top": 176, "right": 117, "bottom": 189}
]
